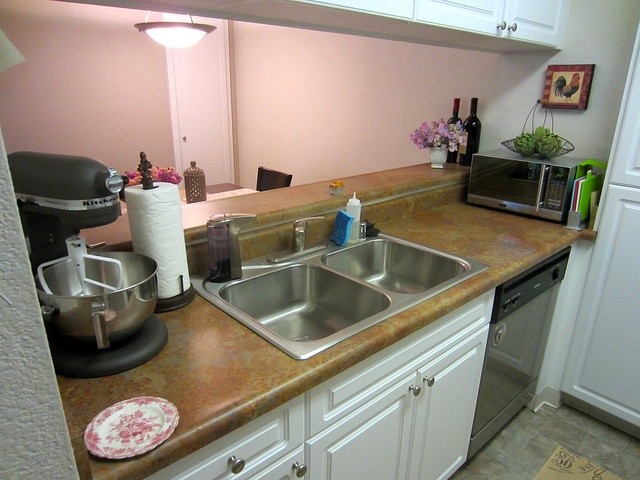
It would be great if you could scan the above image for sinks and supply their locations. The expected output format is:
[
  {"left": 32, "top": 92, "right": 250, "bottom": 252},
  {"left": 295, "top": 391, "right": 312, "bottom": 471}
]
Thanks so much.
[
  {"left": 304, "top": 231, "right": 490, "bottom": 302},
  {"left": 189, "top": 261, "right": 395, "bottom": 361}
]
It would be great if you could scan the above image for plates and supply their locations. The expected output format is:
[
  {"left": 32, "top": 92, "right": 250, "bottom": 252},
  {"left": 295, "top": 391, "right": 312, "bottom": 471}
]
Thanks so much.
[{"left": 85, "top": 397, "right": 179, "bottom": 459}]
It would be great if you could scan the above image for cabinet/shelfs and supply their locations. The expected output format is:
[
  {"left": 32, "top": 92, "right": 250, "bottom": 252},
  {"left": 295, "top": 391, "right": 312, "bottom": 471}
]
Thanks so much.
[
  {"left": 415, "top": 1, "right": 571, "bottom": 52},
  {"left": 145, "top": 389, "right": 308, "bottom": 478},
  {"left": 316, "top": 0, "right": 414, "bottom": 23},
  {"left": 308, "top": 289, "right": 496, "bottom": 480},
  {"left": 562, "top": 26, "right": 640, "bottom": 438}
]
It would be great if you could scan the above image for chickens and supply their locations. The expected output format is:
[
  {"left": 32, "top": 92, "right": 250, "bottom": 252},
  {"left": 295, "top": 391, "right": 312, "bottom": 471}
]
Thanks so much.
[{"left": 553, "top": 73, "right": 581, "bottom": 102}]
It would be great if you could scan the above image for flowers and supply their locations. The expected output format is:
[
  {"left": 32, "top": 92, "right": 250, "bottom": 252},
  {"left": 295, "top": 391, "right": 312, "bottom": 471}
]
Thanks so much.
[{"left": 409, "top": 119, "right": 468, "bottom": 155}]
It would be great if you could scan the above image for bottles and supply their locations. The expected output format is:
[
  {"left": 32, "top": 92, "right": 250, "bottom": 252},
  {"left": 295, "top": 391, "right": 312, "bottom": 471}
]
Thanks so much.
[
  {"left": 460, "top": 98, "right": 481, "bottom": 166},
  {"left": 447, "top": 98, "right": 461, "bottom": 163}
]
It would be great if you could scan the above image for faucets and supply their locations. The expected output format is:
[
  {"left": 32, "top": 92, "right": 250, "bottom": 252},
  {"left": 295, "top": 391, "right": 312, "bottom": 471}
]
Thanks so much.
[{"left": 290, "top": 217, "right": 325, "bottom": 254}]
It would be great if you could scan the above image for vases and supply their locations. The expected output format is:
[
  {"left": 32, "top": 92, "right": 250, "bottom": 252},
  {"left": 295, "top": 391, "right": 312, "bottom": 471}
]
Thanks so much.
[{"left": 430, "top": 147, "right": 449, "bottom": 169}]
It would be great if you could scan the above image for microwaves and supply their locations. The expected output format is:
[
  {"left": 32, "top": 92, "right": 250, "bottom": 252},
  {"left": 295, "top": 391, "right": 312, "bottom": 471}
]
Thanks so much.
[{"left": 468, "top": 145, "right": 590, "bottom": 224}]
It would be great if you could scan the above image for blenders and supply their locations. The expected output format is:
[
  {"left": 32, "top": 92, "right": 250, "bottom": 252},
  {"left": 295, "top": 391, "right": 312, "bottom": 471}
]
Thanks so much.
[{"left": 7, "top": 147, "right": 167, "bottom": 377}]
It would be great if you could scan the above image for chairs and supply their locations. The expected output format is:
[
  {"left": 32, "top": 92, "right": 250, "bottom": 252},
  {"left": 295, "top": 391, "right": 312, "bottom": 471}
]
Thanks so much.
[{"left": 256, "top": 168, "right": 293, "bottom": 190}]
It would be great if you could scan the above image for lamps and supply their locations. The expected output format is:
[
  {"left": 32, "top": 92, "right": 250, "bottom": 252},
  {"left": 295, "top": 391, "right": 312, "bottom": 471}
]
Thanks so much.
[{"left": 132, "top": 6, "right": 217, "bottom": 49}]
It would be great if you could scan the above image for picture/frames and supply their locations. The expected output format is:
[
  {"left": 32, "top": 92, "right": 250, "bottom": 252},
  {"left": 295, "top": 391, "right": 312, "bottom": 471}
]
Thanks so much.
[{"left": 541, "top": 63, "right": 595, "bottom": 110}]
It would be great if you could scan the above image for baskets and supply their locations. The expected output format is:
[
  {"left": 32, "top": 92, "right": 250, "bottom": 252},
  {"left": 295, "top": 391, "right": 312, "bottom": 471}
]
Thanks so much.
[{"left": 502, "top": 132, "right": 574, "bottom": 159}]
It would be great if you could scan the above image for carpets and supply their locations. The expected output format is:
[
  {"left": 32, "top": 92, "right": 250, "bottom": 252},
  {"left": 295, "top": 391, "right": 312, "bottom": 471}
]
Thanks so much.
[{"left": 532, "top": 445, "right": 625, "bottom": 480}]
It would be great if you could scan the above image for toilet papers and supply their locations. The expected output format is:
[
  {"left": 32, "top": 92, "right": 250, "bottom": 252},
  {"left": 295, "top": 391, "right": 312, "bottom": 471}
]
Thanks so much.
[{"left": 119, "top": 181, "right": 192, "bottom": 300}]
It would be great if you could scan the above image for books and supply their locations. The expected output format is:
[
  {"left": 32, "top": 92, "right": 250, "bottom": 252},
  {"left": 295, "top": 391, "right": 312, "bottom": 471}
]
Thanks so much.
[{"left": 570, "top": 171, "right": 603, "bottom": 221}]
